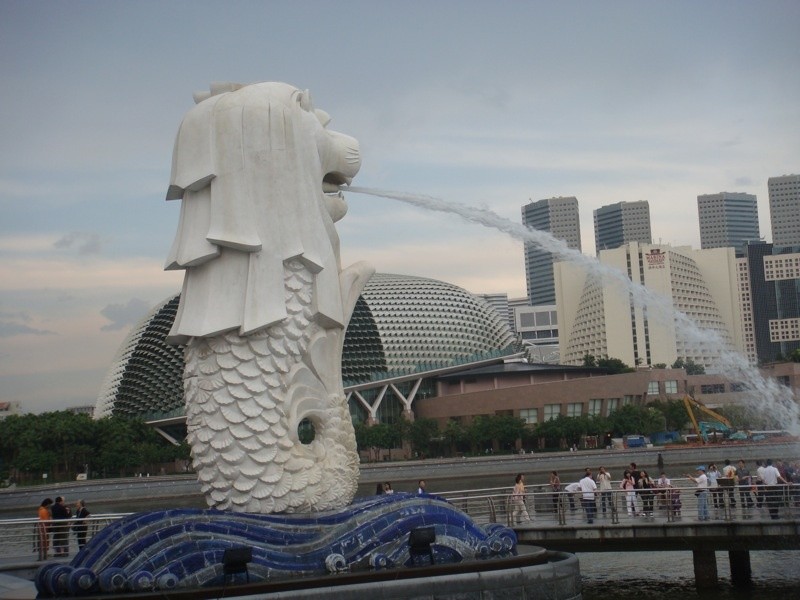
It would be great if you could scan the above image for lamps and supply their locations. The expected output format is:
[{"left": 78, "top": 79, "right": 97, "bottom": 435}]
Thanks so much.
[
  {"left": 220, "top": 546, "right": 254, "bottom": 585},
  {"left": 407, "top": 526, "right": 436, "bottom": 567}
]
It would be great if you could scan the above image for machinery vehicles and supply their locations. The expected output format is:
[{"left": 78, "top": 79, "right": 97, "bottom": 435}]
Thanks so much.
[{"left": 683, "top": 392, "right": 756, "bottom": 446}]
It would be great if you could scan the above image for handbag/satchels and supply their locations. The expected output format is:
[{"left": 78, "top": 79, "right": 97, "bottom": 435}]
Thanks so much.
[{"left": 694, "top": 491, "right": 702, "bottom": 497}]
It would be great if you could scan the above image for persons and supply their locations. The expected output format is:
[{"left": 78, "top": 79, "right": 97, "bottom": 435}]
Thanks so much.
[
  {"left": 509, "top": 472, "right": 531, "bottom": 526},
  {"left": 376, "top": 481, "right": 393, "bottom": 495},
  {"left": 37, "top": 498, "right": 54, "bottom": 560},
  {"left": 71, "top": 499, "right": 90, "bottom": 551},
  {"left": 549, "top": 470, "right": 562, "bottom": 513},
  {"left": 51, "top": 496, "right": 73, "bottom": 557},
  {"left": 417, "top": 480, "right": 425, "bottom": 494},
  {"left": 564, "top": 458, "right": 800, "bottom": 522}
]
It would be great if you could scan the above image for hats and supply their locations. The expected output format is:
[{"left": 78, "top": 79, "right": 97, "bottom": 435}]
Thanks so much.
[{"left": 696, "top": 466, "right": 705, "bottom": 471}]
